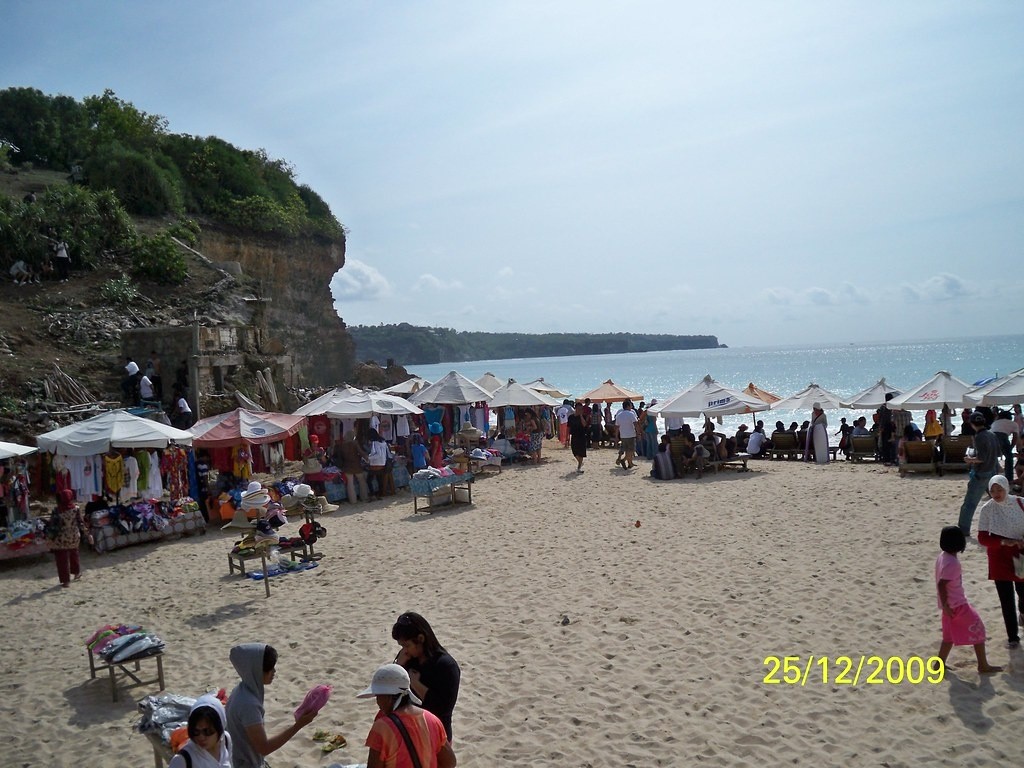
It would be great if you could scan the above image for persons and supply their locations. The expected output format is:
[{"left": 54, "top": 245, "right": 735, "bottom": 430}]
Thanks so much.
[
  {"left": 935, "top": 526, "right": 1005, "bottom": 674},
  {"left": 47, "top": 489, "right": 94, "bottom": 587},
  {"left": 958, "top": 413, "right": 1000, "bottom": 535},
  {"left": 297, "top": 421, "right": 446, "bottom": 509},
  {"left": 976, "top": 474, "right": 1024, "bottom": 650},
  {"left": 168, "top": 695, "right": 234, "bottom": 768},
  {"left": 518, "top": 399, "right": 1024, "bottom": 480},
  {"left": 224, "top": 643, "right": 318, "bottom": 768},
  {"left": 391, "top": 611, "right": 461, "bottom": 747},
  {"left": 9, "top": 236, "right": 69, "bottom": 287},
  {"left": 117, "top": 349, "right": 194, "bottom": 428},
  {"left": 355, "top": 664, "right": 458, "bottom": 768}
]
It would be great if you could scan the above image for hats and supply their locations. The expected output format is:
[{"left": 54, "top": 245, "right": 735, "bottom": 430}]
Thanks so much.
[
  {"left": 470, "top": 448, "right": 486, "bottom": 460},
  {"left": 738, "top": 424, "right": 748, "bottom": 429},
  {"left": 428, "top": 422, "right": 443, "bottom": 434},
  {"left": 221, "top": 481, "right": 339, "bottom": 556},
  {"left": 813, "top": 402, "right": 821, "bottom": 410},
  {"left": 645, "top": 398, "right": 660, "bottom": 405},
  {"left": 460, "top": 421, "right": 476, "bottom": 434},
  {"left": 355, "top": 664, "right": 422, "bottom": 710}
]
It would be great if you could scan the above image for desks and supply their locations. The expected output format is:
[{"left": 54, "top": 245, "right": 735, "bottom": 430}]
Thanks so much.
[
  {"left": 91, "top": 510, "right": 207, "bottom": 556},
  {"left": 310, "top": 464, "right": 411, "bottom": 505},
  {"left": 87, "top": 643, "right": 166, "bottom": 702},
  {"left": 228, "top": 545, "right": 309, "bottom": 578},
  {"left": 144, "top": 733, "right": 175, "bottom": 768},
  {"left": 0, "top": 540, "right": 50, "bottom": 560},
  {"left": 470, "top": 457, "right": 502, "bottom": 476},
  {"left": 409, "top": 472, "right": 475, "bottom": 515}
]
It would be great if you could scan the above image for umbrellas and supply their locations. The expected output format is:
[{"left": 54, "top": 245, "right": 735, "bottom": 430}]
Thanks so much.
[
  {"left": 475, "top": 373, "right": 572, "bottom": 409},
  {"left": 408, "top": 370, "right": 493, "bottom": 447},
  {"left": 885, "top": 370, "right": 978, "bottom": 436},
  {"left": 839, "top": 378, "right": 906, "bottom": 410},
  {"left": 0, "top": 409, "right": 305, "bottom": 466},
  {"left": 963, "top": 367, "right": 1024, "bottom": 405},
  {"left": 736, "top": 383, "right": 783, "bottom": 428},
  {"left": 575, "top": 379, "right": 643, "bottom": 403},
  {"left": 771, "top": 384, "right": 844, "bottom": 410},
  {"left": 646, "top": 374, "right": 770, "bottom": 432},
  {"left": 290, "top": 375, "right": 427, "bottom": 417}
]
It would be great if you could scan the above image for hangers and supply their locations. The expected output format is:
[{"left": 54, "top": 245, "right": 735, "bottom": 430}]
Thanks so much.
[{"left": 105, "top": 449, "right": 120, "bottom": 456}]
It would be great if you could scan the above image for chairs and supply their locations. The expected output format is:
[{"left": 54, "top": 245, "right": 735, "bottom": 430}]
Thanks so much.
[
  {"left": 938, "top": 434, "right": 972, "bottom": 477},
  {"left": 791, "top": 431, "right": 839, "bottom": 462},
  {"left": 766, "top": 432, "right": 798, "bottom": 461},
  {"left": 694, "top": 440, "right": 752, "bottom": 475},
  {"left": 849, "top": 434, "right": 879, "bottom": 464},
  {"left": 605, "top": 423, "right": 622, "bottom": 448},
  {"left": 900, "top": 441, "right": 936, "bottom": 479}
]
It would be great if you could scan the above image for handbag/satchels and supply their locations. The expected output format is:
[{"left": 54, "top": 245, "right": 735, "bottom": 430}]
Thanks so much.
[
  {"left": 47, "top": 508, "right": 65, "bottom": 541},
  {"left": 302, "top": 448, "right": 321, "bottom": 474},
  {"left": 294, "top": 685, "right": 332, "bottom": 725},
  {"left": 693, "top": 444, "right": 711, "bottom": 459}
]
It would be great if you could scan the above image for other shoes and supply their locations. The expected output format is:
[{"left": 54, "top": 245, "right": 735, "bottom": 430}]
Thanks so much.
[
  {"left": 313, "top": 728, "right": 334, "bottom": 740},
  {"left": 62, "top": 582, "right": 69, "bottom": 587},
  {"left": 620, "top": 460, "right": 627, "bottom": 470},
  {"left": 322, "top": 734, "right": 348, "bottom": 752},
  {"left": 616, "top": 459, "right": 620, "bottom": 465},
  {"left": 74, "top": 572, "right": 82, "bottom": 582},
  {"left": 628, "top": 464, "right": 633, "bottom": 468},
  {"left": 1019, "top": 613, "right": 1024, "bottom": 625},
  {"left": 1007, "top": 641, "right": 1018, "bottom": 650},
  {"left": 577, "top": 470, "right": 584, "bottom": 473}
]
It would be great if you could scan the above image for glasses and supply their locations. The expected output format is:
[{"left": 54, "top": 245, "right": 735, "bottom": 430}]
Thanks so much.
[
  {"left": 398, "top": 614, "right": 422, "bottom": 632},
  {"left": 190, "top": 727, "right": 219, "bottom": 737}
]
